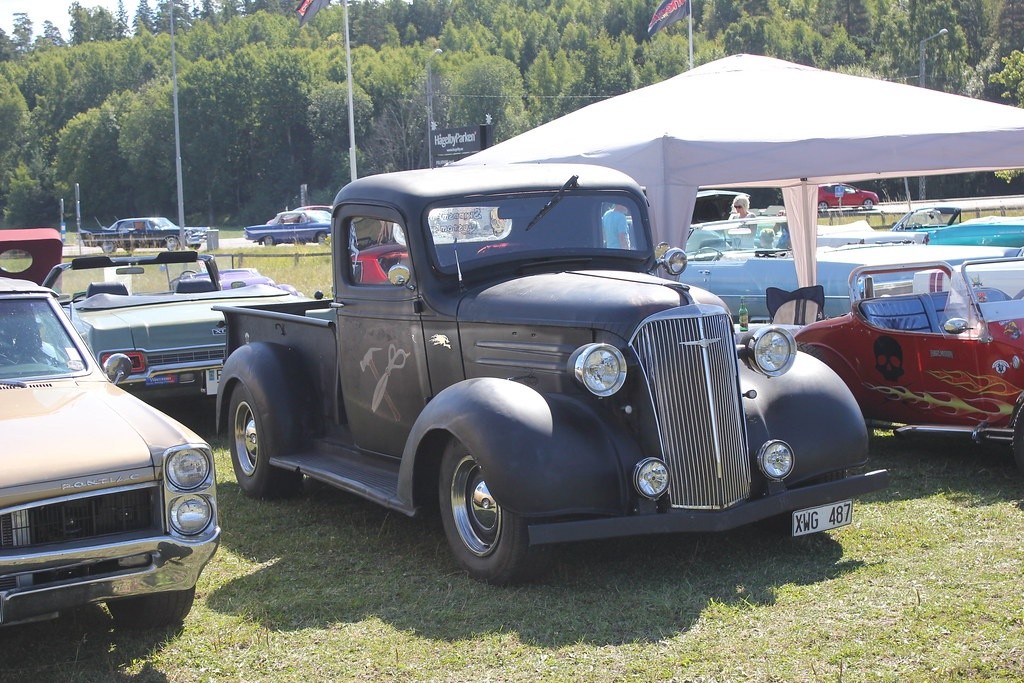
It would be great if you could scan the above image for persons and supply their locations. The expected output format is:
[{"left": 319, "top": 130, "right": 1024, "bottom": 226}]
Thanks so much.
[
  {"left": 759, "top": 228, "right": 775, "bottom": 250},
  {"left": 728, "top": 195, "right": 757, "bottom": 249},
  {"left": 602, "top": 203, "right": 629, "bottom": 250},
  {"left": 774, "top": 209, "right": 791, "bottom": 249}
]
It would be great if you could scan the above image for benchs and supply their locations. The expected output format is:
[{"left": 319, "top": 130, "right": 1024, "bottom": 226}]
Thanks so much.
[{"left": 859, "top": 288, "right": 1007, "bottom": 333}]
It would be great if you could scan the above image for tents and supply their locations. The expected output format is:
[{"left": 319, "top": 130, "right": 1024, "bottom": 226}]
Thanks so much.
[{"left": 442, "top": 52, "right": 1024, "bottom": 282}]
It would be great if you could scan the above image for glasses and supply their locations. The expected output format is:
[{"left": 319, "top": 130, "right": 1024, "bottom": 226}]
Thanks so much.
[{"left": 734, "top": 205, "right": 745, "bottom": 210}]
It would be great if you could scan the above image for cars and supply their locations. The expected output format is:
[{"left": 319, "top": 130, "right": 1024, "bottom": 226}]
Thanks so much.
[
  {"left": 817, "top": 182, "right": 880, "bottom": 215},
  {"left": 38, "top": 248, "right": 310, "bottom": 416},
  {"left": 85, "top": 216, "right": 210, "bottom": 251},
  {"left": 651, "top": 190, "right": 1024, "bottom": 477},
  {"left": 0, "top": 274, "right": 223, "bottom": 645},
  {"left": 265, "top": 205, "right": 333, "bottom": 226},
  {"left": 243, "top": 210, "right": 332, "bottom": 248}
]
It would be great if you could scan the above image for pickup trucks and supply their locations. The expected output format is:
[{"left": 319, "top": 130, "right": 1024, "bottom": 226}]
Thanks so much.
[{"left": 210, "top": 161, "right": 893, "bottom": 588}]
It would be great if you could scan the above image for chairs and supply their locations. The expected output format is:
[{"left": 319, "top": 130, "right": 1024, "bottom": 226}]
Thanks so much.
[
  {"left": 475, "top": 242, "right": 531, "bottom": 259},
  {"left": 86, "top": 281, "right": 129, "bottom": 298},
  {"left": 766, "top": 285, "right": 824, "bottom": 325},
  {"left": 700, "top": 238, "right": 724, "bottom": 249},
  {"left": 177, "top": 279, "right": 218, "bottom": 293},
  {"left": 0, "top": 303, "right": 42, "bottom": 350},
  {"left": 351, "top": 243, "right": 410, "bottom": 285}
]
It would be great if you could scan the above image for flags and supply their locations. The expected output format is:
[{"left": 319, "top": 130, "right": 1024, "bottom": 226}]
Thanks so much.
[
  {"left": 648, "top": 0, "right": 691, "bottom": 35},
  {"left": 294, "top": 0, "right": 330, "bottom": 27}
]
